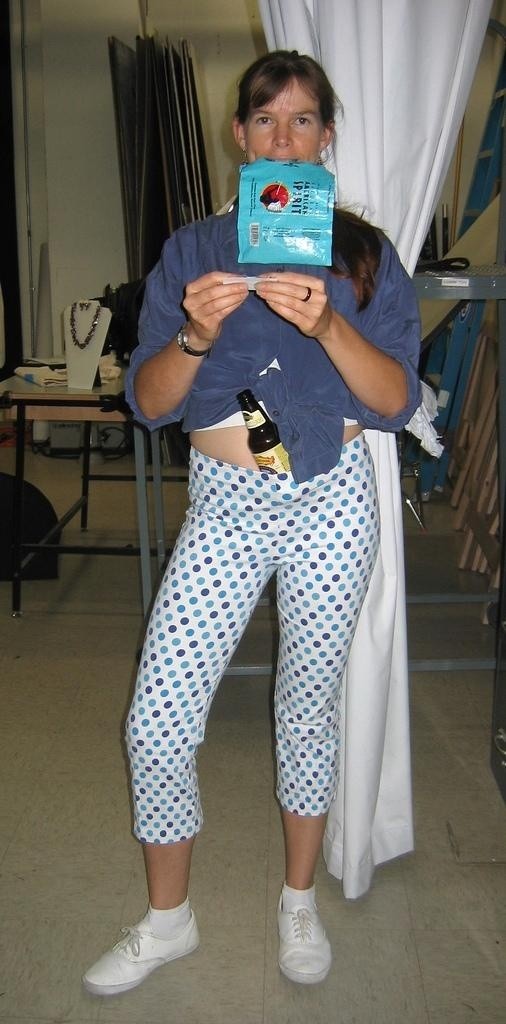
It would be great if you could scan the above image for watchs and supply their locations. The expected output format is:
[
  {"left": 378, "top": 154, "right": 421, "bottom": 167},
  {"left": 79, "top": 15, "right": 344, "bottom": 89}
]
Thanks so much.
[{"left": 175, "top": 317, "right": 214, "bottom": 358}]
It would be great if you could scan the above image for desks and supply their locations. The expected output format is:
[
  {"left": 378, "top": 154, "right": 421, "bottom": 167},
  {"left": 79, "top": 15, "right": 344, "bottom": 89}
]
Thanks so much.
[{"left": 0, "top": 361, "right": 156, "bottom": 636}]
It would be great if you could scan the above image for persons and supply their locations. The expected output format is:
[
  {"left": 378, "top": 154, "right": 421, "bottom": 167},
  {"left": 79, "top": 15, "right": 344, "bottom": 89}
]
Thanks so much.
[{"left": 81, "top": 46, "right": 419, "bottom": 996}]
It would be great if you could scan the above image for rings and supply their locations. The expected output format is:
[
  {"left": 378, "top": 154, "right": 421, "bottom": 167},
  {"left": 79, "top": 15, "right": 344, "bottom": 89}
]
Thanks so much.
[{"left": 299, "top": 282, "right": 316, "bottom": 303}]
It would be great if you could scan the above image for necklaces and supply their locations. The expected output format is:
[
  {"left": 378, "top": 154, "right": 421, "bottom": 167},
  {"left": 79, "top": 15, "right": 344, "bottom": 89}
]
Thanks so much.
[{"left": 71, "top": 300, "right": 100, "bottom": 351}]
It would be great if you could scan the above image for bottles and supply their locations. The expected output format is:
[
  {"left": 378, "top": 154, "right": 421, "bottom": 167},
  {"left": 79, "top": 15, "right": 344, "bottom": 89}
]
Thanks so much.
[{"left": 235, "top": 387, "right": 293, "bottom": 474}]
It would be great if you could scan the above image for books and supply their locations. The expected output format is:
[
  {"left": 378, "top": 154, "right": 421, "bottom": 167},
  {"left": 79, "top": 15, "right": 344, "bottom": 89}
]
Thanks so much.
[{"left": 14, "top": 352, "right": 68, "bottom": 386}]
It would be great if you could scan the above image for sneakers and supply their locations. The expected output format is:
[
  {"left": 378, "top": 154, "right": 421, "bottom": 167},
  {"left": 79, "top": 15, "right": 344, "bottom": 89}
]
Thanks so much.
[
  {"left": 82, "top": 912, "right": 199, "bottom": 996},
  {"left": 276, "top": 892, "right": 333, "bottom": 986}
]
